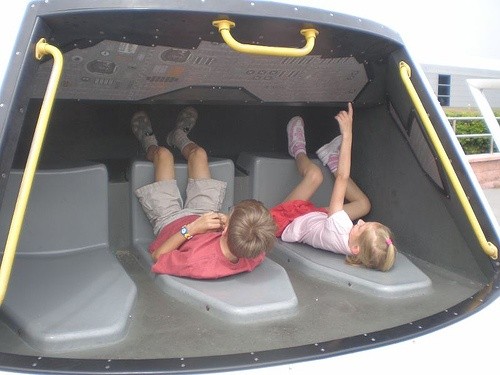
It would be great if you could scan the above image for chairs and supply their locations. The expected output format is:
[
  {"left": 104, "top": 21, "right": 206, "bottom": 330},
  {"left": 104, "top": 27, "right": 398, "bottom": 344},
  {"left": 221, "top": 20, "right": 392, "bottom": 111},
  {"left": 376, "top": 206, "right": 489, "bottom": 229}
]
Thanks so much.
[
  {"left": 129, "top": 154, "right": 300, "bottom": 324},
  {"left": 237, "top": 149, "right": 432, "bottom": 300},
  {"left": 0, "top": 162, "right": 139, "bottom": 354}
]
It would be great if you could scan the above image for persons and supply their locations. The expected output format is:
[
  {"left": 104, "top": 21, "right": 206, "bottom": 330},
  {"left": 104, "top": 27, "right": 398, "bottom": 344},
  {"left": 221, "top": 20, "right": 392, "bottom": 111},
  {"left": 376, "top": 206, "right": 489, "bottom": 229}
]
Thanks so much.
[
  {"left": 130, "top": 107, "right": 275, "bottom": 279},
  {"left": 268, "top": 101, "right": 397, "bottom": 272}
]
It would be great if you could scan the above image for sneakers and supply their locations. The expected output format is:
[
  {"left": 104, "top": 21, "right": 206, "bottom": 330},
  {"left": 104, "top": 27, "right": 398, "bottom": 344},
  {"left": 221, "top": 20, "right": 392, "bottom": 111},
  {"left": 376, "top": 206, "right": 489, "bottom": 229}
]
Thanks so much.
[
  {"left": 286, "top": 115, "right": 306, "bottom": 157},
  {"left": 130, "top": 111, "right": 156, "bottom": 149},
  {"left": 165, "top": 106, "right": 197, "bottom": 147},
  {"left": 316, "top": 134, "right": 344, "bottom": 164}
]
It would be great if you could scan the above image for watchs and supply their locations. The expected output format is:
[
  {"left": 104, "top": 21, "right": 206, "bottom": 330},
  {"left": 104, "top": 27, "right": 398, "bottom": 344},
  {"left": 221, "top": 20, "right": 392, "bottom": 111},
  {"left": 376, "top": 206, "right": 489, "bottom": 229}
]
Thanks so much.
[{"left": 180, "top": 225, "right": 193, "bottom": 240}]
